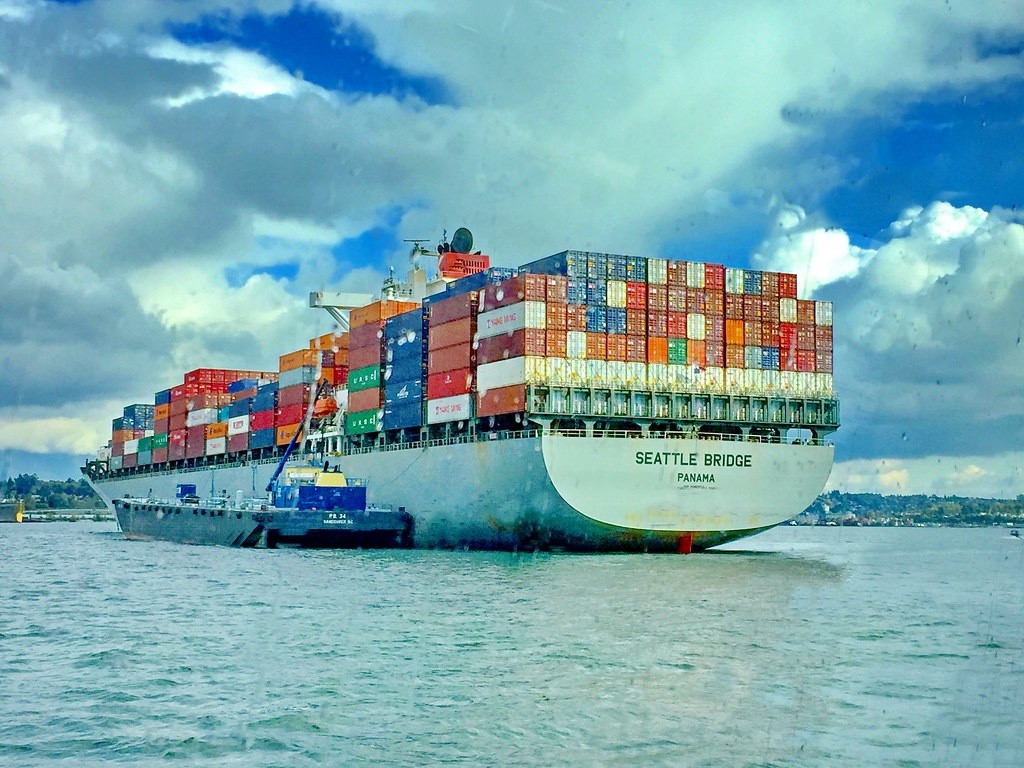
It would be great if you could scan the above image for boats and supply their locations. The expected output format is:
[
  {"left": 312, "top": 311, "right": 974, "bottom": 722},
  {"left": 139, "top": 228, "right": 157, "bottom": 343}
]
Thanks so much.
[
  {"left": 80, "top": 228, "right": 835, "bottom": 555},
  {"left": 112, "top": 378, "right": 415, "bottom": 548}
]
